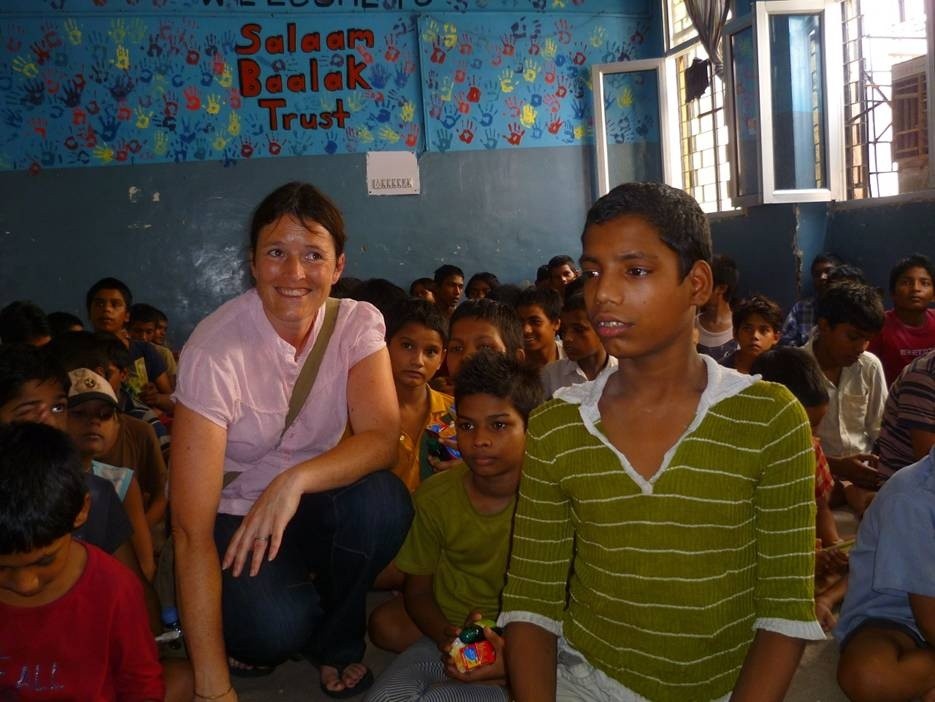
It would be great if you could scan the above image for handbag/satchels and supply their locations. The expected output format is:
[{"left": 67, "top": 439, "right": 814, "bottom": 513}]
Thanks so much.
[{"left": 155, "top": 535, "right": 178, "bottom": 624}]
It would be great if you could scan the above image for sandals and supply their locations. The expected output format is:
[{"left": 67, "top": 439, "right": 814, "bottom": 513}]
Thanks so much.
[
  {"left": 315, "top": 661, "right": 374, "bottom": 699},
  {"left": 228, "top": 658, "right": 255, "bottom": 676}
]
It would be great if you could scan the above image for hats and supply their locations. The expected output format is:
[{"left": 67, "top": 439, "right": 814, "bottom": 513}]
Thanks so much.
[{"left": 64, "top": 367, "right": 118, "bottom": 406}]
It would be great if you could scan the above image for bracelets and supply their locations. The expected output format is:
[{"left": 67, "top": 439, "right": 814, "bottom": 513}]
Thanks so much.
[{"left": 194, "top": 686, "right": 233, "bottom": 700}]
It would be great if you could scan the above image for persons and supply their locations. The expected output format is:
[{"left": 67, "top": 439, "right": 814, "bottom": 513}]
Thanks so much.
[
  {"left": 497, "top": 182, "right": 828, "bottom": 702},
  {"left": 0, "top": 278, "right": 195, "bottom": 702},
  {"left": 695, "top": 254, "right": 935, "bottom": 630},
  {"left": 0, "top": 418, "right": 166, "bottom": 702},
  {"left": 364, "top": 348, "right": 545, "bottom": 702},
  {"left": 171, "top": 181, "right": 415, "bottom": 702},
  {"left": 835, "top": 442, "right": 935, "bottom": 702},
  {"left": 329, "top": 255, "right": 618, "bottom": 651}
]
